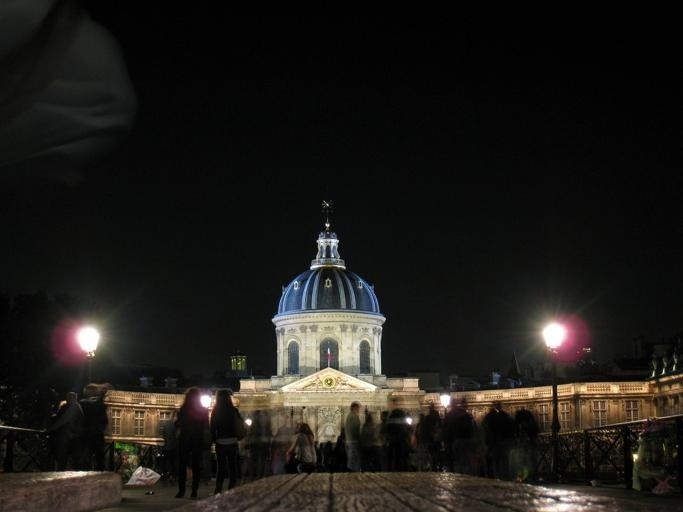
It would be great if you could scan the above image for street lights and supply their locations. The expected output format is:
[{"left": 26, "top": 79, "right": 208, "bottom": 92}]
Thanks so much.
[
  {"left": 198, "top": 392, "right": 211, "bottom": 413},
  {"left": 536, "top": 317, "right": 571, "bottom": 485},
  {"left": 73, "top": 321, "right": 105, "bottom": 387},
  {"left": 438, "top": 393, "right": 452, "bottom": 417}
]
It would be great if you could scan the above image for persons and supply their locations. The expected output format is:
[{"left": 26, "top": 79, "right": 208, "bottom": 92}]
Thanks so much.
[
  {"left": 173, "top": 387, "right": 540, "bottom": 498},
  {"left": 42, "top": 382, "right": 110, "bottom": 472}
]
